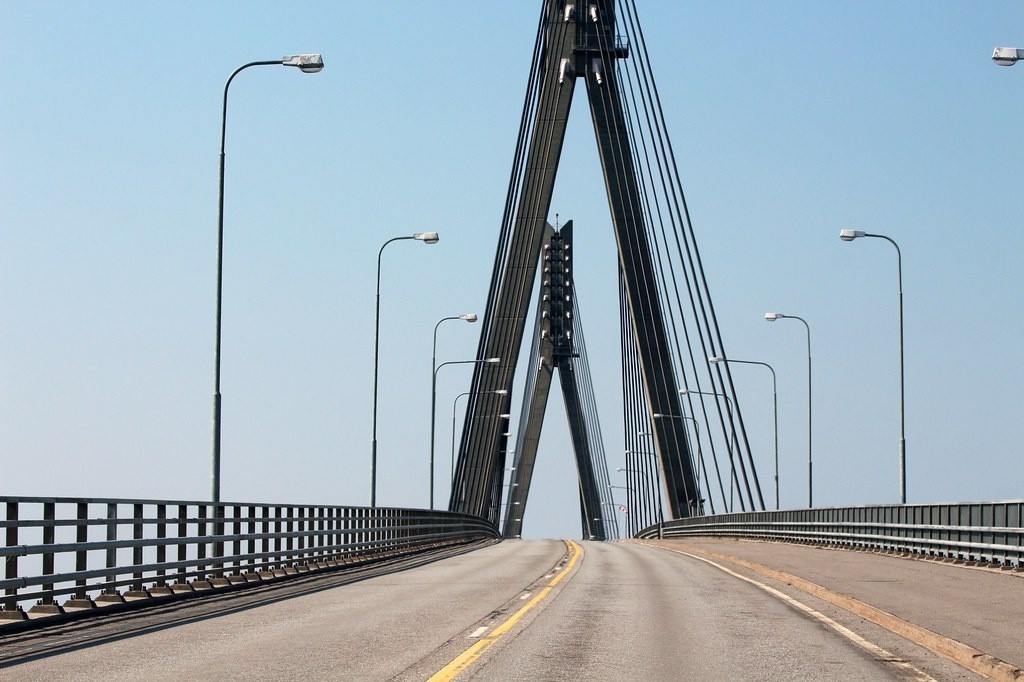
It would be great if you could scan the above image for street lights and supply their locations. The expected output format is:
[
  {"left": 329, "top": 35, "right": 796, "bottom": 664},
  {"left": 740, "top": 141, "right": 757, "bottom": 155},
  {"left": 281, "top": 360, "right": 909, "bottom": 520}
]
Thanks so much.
[
  {"left": 708, "top": 356, "right": 782, "bottom": 511},
  {"left": 678, "top": 389, "right": 734, "bottom": 514},
  {"left": 652, "top": 413, "right": 700, "bottom": 518},
  {"left": 370, "top": 231, "right": 443, "bottom": 509},
  {"left": 763, "top": 311, "right": 814, "bottom": 509},
  {"left": 606, "top": 432, "right": 661, "bottom": 541},
  {"left": 212, "top": 52, "right": 327, "bottom": 506},
  {"left": 426, "top": 312, "right": 500, "bottom": 508},
  {"left": 837, "top": 227, "right": 907, "bottom": 506},
  {"left": 592, "top": 501, "right": 628, "bottom": 540},
  {"left": 449, "top": 389, "right": 509, "bottom": 512}
]
